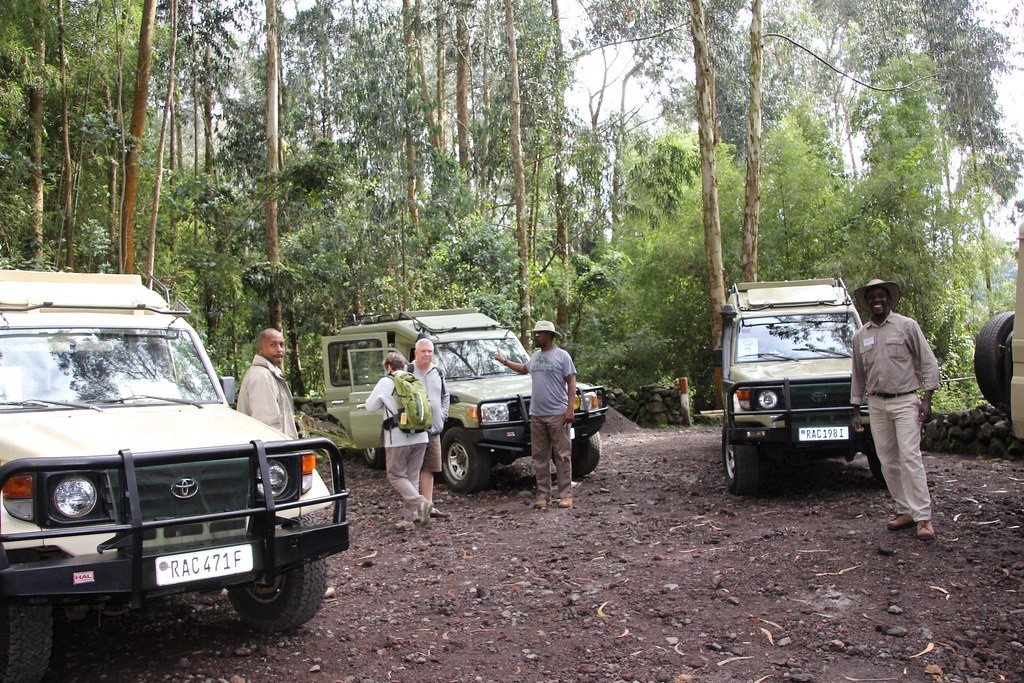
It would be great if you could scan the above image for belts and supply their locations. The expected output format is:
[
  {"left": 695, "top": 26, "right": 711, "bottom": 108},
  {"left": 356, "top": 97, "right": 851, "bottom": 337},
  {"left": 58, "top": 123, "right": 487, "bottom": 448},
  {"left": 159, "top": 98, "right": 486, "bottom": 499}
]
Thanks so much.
[{"left": 871, "top": 390, "right": 916, "bottom": 398}]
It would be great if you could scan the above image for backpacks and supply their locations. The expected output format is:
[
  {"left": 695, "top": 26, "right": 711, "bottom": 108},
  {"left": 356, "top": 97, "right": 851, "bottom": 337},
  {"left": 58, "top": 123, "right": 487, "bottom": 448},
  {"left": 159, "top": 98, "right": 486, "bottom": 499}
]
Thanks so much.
[{"left": 383, "top": 371, "right": 433, "bottom": 434}]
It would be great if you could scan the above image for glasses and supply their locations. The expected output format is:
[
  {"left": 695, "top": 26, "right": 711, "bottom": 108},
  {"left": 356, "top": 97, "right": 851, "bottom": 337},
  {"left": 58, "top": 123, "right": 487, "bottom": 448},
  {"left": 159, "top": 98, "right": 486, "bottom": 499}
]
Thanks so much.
[{"left": 534, "top": 332, "right": 546, "bottom": 337}]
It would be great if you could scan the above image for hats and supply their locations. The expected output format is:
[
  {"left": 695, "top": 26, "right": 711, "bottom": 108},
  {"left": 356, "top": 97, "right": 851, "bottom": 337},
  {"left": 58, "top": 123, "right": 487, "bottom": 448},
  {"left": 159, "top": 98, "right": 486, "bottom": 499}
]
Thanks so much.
[
  {"left": 855, "top": 279, "right": 901, "bottom": 313},
  {"left": 528, "top": 321, "right": 562, "bottom": 339}
]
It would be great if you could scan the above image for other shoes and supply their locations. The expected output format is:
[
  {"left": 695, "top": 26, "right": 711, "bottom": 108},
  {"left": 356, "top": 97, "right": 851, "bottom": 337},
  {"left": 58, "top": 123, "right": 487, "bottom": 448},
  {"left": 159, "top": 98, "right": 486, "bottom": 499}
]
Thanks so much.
[
  {"left": 430, "top": 509, "right": 448, "bottom": 517},
  {"left": 395, "top": 520, "right": 414, "bottom": 531},
  {"left": 420, "top": 501, "right": 430, "bottom": 526}
]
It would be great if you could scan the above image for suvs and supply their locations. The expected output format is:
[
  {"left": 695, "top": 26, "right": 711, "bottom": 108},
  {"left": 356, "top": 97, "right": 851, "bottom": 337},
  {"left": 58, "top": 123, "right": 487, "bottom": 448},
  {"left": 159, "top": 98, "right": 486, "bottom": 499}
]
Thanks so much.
[
  {"left": 710, "top": 278, "right": 886, "bottom": 495},
  {"left": 974, "top": 223, "right": 1024, "bottom": 440},
  {"left": 293, "top": 307, "right": 605, "bottom": 498},
  {"left": 0, "top": 270, "right": 347, "bottom": 683}
]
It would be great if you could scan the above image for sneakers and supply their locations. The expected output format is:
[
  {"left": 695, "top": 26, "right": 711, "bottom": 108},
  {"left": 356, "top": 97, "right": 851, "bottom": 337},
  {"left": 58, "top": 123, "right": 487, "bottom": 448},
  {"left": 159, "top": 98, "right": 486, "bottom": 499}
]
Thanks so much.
[
  {"left": 887, "top": 514, "right": 915, "bottom": 530},
  {"left": 558, "top": 497, "right": 572, "bottom": 508},
  {"left": 916, "top": 520, "right": 936, "bottom": 540},
  {"left": 534, "top": 499, "right": 547, "bottom": 509}
]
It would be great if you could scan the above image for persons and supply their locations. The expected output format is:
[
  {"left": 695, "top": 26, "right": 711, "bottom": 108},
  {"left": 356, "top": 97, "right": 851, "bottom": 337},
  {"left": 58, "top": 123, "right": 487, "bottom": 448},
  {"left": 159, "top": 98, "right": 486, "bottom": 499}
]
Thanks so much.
[
  {"left": 404, "top": 338, "right": 450, "bottom": 523},
  {"left": 365, "top": 352, "right": 434, "bottom": 531},
  {"left": 495, "top": 320, "right": 578, "bottom": 509},
  {"left": 849, "top": 278, "right": 940, "bottom": 540},
  {"left": 237, "top": 327, "right": 299, "bottom": 440}
]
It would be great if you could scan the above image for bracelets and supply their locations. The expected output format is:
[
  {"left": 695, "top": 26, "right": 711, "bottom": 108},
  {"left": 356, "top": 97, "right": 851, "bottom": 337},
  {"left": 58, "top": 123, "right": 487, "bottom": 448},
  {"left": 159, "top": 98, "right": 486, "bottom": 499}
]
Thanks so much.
[{"left": 504, "top": 358, "right": 506, "bottom": 366}]
